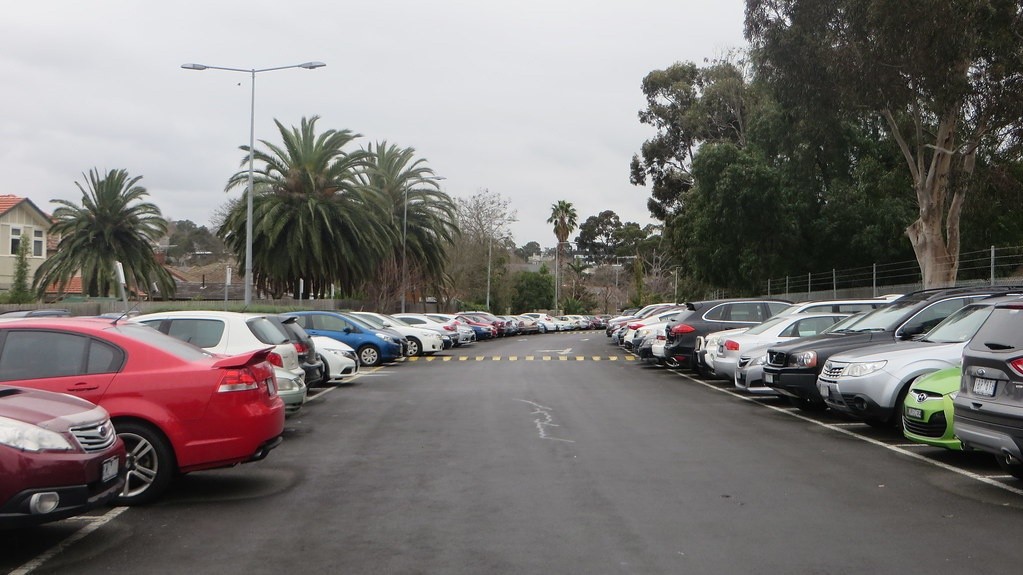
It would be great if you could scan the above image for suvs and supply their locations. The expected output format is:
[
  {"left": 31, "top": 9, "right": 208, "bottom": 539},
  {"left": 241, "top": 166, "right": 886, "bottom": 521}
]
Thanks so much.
[
  {"left": 761, "top": 286, "right": 1023, "bottom": 422},
  {"left": 816, "top": 290, "right": 1023, "bottom": 430}
]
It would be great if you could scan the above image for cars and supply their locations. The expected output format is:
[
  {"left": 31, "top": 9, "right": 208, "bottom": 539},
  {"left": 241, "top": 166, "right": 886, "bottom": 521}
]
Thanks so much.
[
  {"left": 0, "top": 315, "right": 285, "bottom": 506},
  {"left": 310, "top": 336, "right": 360, "bottom": 382},
  {"left": 126, "top": 311, "right": 307, "bottom": 416},
  {"left": 390, "top": 314, "right": 498, "bottom": 349},
  {"left": 0, "top": 385, "right": 126, "bottom": 525},
  {"left": 460, "top": 311, "right": 621, "bottom": 334},
  {"left": 338, "top": 312, "right": 408, "bottom": 355},
  {"left": 606, "top": 298, "right": 749, "bottom": 369},
  {"left": 351, "top": 311, "right": 444, "bottom": 357},
  {"left": 274, "top": 314, "right": 325, "bottom": 395},
  {"left": 282, "top": 311, "right": 404, "bottom": 367},
  {"left": 663, "top": 294, "right": 920, "bottom": 396},
  {"left": 901, "top": 299, "right": 1023, "bottom": 479}
]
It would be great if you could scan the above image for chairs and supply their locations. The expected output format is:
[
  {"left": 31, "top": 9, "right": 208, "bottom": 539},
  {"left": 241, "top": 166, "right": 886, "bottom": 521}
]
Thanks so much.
[{"left": 748, "top": 305, "right": 760, "bottom": 321}]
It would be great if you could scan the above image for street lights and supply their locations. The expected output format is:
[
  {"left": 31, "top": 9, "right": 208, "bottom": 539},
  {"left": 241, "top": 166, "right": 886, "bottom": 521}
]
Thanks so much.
[
  {"left": 616, "top": 257, "right": 637, "bottom": 315},
  {"left": 487, "top": 218, "right": 519, "bottom": 310},
  {"left": 182, "top": 62, "right": 447, "bottom": 312},
  {"left": 675, "top": 266, "right": 685, "bottom": 302},
  {"left": 555, "top": 242, "right": 578, "bottom": 315}
]
